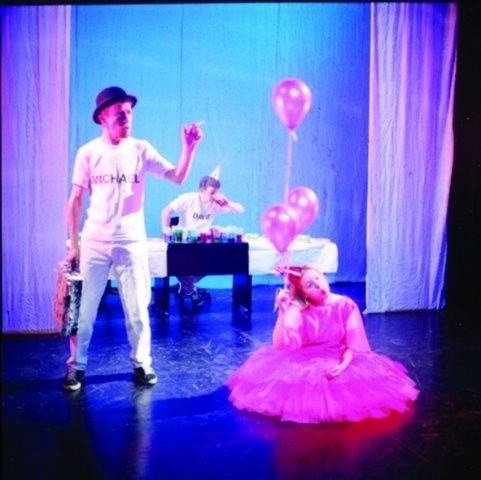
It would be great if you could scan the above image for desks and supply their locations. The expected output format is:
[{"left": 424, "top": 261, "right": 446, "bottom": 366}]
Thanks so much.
[{"left": 65, "top": 237, "right": 338, "bottom": 321}]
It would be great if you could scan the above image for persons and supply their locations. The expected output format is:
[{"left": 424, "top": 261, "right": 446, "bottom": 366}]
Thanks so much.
[
  {"left": 160, "top": 164, "right": 245, "bottom": 304},
  {"left": 63, "top": 86, "right": 203, "bottom": 390},
  {"left": 225, "top": 265, "right": 420, "bottom": 425}
]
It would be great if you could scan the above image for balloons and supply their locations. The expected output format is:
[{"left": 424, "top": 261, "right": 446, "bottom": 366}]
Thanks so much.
[
  {"left": 270, "top": 78, "right": 312, "bottom": 206},
  {"left": 288, "top": 188, "right": 319, "bottom": 282},
  {"left": 261, "top": 203, "right": 299, "bottom": 290}
]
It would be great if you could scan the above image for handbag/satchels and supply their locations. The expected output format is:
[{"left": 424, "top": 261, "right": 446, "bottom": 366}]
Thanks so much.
[{"left": 53, "top": 261, "right": 83, "bottom": 338}]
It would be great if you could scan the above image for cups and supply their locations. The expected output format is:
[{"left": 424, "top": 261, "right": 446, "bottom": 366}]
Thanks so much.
[{"left": 167, "top": 225, "right": 243, "bottom": 244}]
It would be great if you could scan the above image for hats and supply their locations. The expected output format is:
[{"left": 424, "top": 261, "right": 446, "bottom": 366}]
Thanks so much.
[
  {"left": 273, "top": 265, "right": 308, "bottom": 290},
  {"left": 92, "top": 87, "right": 137, "bottom": 124},
  {"left": 210, "top": 165, "right": 220, "bottom": 181}
]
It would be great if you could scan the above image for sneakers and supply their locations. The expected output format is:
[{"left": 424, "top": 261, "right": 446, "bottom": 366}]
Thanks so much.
[
  {"left": 63, "top": 366, "right": 84, "bottom": 391},
  {"left": 133, "top": 367, "right": 157, "bottom": 385}
]
